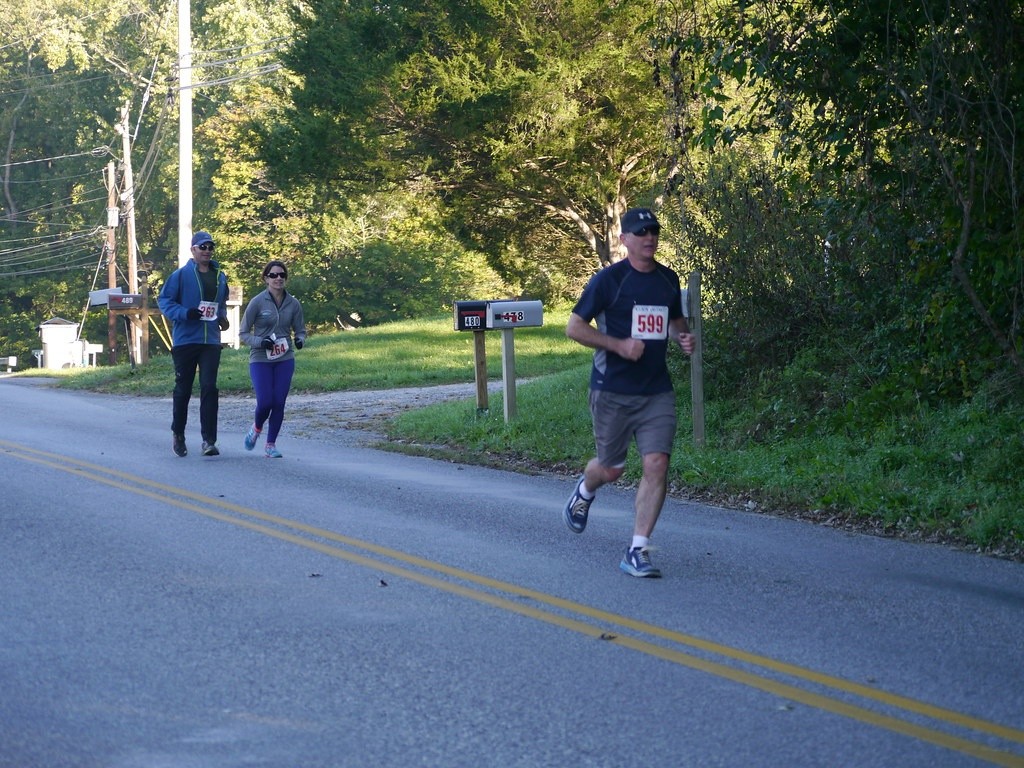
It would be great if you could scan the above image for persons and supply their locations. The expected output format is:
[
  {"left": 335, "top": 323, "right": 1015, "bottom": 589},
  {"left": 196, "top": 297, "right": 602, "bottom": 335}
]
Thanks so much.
[
  {"left": 563, "top": 209, "right": 695, "bottom": 578},
  {"left": 239, "top": 261, "right": 307, "bottom": 457},
  {"left": 157, "top": 232, "right": 229, "bottom": 456}
]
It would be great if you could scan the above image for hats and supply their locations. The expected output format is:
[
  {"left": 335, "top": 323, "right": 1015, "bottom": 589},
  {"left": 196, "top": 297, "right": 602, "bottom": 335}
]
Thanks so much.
[
  {"left": 192, "top": 232, "right": 215, "bottom": 245},
  {"left": 621, "top": 208, "right": 660, "bottom": 233}
]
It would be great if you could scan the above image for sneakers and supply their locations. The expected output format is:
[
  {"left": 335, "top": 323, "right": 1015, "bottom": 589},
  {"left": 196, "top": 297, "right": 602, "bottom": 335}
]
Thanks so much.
[
  {"left": 265, "top": 442, "right": 281, "bottom": 458},
  {"left": 172, "top": 432, "right": 188, "bottom": 457},
  {"left": 243, "top": 423, "right": 261, "bottom": 450},
  {"left": 563, "top": 475, "right": 596, "bottom": 533},
  {"left": 201, "top": 442, "right": 219, "bottom": 455},
  {"left": 619, "top": 547, "right": 662, "bottom": 578}
]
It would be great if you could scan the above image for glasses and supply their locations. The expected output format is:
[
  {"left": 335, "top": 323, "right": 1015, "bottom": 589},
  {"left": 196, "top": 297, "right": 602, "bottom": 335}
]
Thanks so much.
[
  {"left": 193, "top": 244, "right": 214, "bottom": 251},
  {"left": 267, "top": 272, "right": 287, "bottom": 278},
  {"left": 633, "top": 226, "right": 659, "bottom": 236}
]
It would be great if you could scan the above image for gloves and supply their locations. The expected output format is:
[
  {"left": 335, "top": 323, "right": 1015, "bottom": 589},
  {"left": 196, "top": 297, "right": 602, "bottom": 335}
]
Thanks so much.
[
  {"left": 187, "top": 308, "right": 203, "bottom": 320},
  {"left": 294, "top": 337, "right": 302, "bottom": 350},
  {"left": 262, "top": 336, "right": 274, "bottom": 350},
  {"left": 218, "top": 319, "right": 229, "bottom": 331}
]
